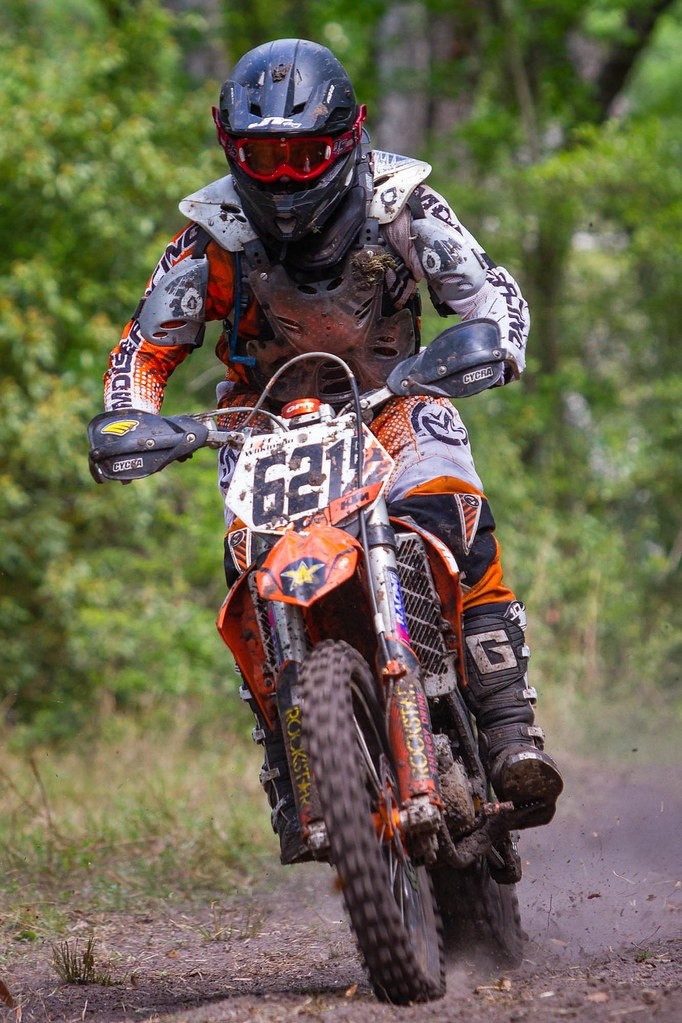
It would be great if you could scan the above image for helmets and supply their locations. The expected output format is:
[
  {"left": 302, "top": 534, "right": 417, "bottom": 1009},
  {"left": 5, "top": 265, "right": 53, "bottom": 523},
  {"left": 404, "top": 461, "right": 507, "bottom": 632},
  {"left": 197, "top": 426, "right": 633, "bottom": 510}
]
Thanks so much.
[{"left": 218, "top": 38, "right": 367, "bottom": 241}]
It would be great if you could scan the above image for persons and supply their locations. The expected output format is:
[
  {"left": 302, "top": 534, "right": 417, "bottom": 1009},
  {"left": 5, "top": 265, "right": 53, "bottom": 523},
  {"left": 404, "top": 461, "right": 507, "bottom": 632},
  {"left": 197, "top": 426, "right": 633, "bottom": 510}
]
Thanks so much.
[{"left": 104, "top": 39, "right": 565, "bottom": 862}]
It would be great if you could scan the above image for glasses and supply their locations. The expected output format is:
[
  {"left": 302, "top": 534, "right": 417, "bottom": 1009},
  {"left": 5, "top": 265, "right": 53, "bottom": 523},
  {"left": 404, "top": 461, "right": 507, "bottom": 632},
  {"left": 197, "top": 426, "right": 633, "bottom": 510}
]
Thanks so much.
[{"left": 220, "top": 133, "right": 338, "bottom": 182}]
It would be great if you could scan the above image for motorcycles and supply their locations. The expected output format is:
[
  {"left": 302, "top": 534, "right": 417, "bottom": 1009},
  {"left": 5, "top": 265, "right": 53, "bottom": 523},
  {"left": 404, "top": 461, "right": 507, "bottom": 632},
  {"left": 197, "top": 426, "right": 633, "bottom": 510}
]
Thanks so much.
[{"left": 85, "top": 317, "right": 551, "bottom": 1006}]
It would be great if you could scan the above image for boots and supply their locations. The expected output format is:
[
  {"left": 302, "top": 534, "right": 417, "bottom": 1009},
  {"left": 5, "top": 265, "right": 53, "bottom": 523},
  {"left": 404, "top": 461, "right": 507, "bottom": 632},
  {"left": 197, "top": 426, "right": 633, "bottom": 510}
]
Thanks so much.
[
  {"left": 233, "top": 660, "right": 313, "bottom": 864},
  {"left": 459, "top": 598, "right": 563, "bottom": 828}
]
